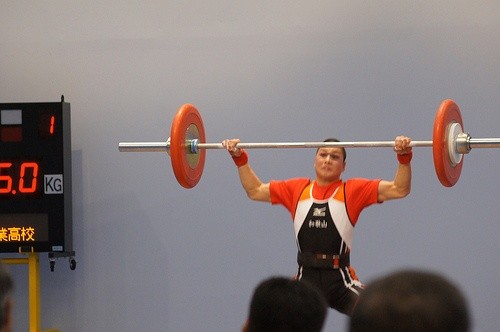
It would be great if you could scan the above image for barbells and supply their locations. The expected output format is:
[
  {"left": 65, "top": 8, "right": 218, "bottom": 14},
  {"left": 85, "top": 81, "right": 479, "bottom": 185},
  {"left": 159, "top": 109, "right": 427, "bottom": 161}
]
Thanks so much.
[{"left": 118, "top": 99, "right": 500, "bottom": 189}]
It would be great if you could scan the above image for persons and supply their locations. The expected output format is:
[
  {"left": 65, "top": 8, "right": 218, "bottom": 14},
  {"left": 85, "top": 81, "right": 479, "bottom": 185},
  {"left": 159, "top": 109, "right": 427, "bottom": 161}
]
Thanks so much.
[
  {"left": 0, "top": 264, "right": 15, "bottom": 332},
  {"left": 222, "top": 136, "right": 412, "bottom": 316},
  {"left": 349, "top": 269, "right": 471, "bottom": 332},
  {"left": 241, "top": 276, "right": 328, "bottom": 332}
]
